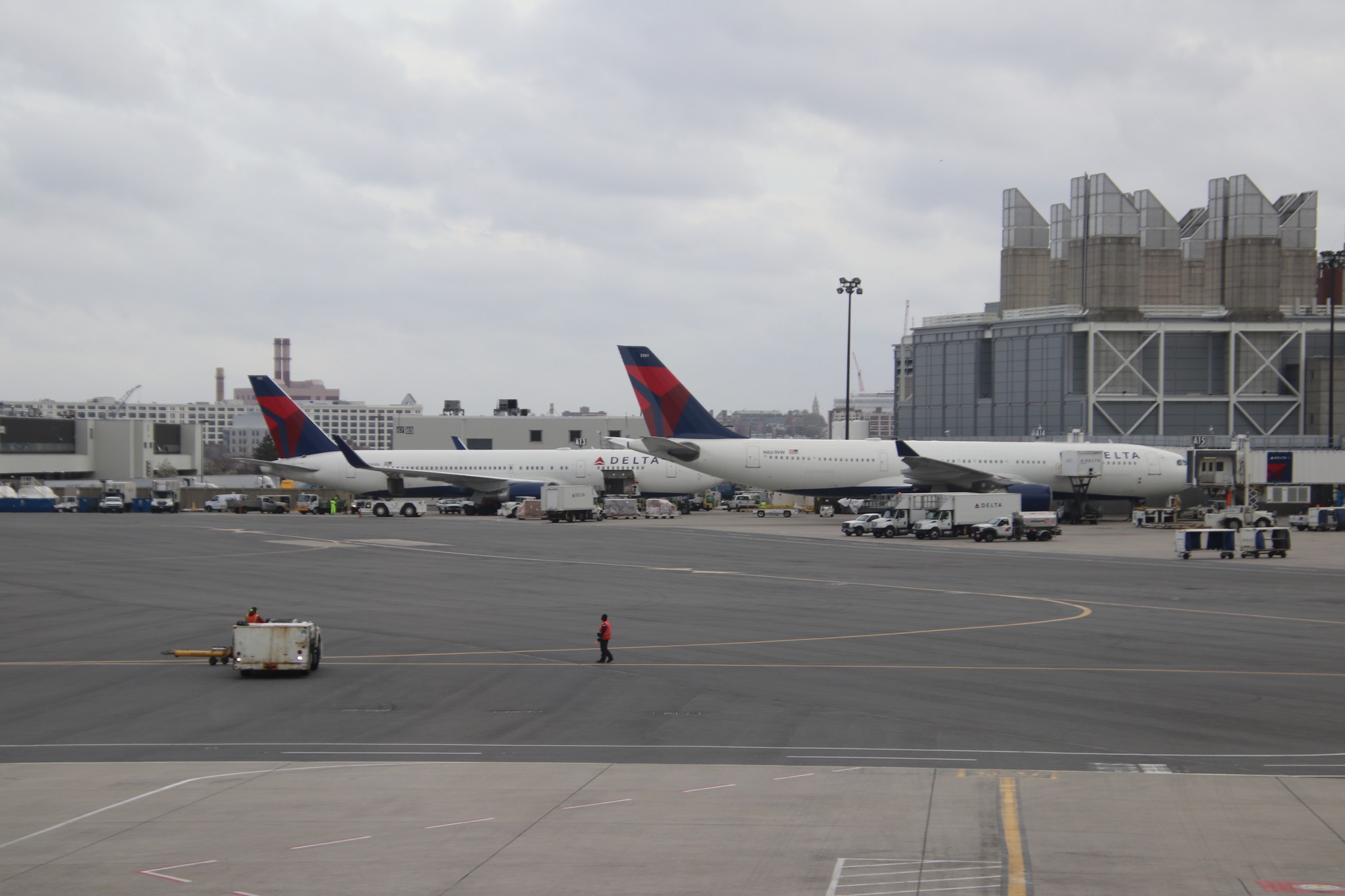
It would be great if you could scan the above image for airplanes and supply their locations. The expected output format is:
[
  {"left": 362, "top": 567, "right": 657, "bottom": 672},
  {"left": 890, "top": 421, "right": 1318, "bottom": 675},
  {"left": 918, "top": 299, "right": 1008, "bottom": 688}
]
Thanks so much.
[
  {"left": 603, "top": 346, "right": 1193, "bottom": 512},
  {"left": 229, "top": 375, "right": 726, "bottom": 516}
]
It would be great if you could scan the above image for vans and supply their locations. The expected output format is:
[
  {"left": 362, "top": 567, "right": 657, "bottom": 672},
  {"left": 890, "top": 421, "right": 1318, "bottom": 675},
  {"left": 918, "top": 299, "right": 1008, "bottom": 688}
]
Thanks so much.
[{"left": 204, "top": 494, "right": 250, "bottom": 513}]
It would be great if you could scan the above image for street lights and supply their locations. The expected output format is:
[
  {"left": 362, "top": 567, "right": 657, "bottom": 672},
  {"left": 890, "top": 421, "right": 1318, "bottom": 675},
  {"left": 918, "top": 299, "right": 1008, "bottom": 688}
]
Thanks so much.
[
  {"left": 1318, "top": 250, "right": 1345, "bottom": 448},
  {"left": 837, "top": 277, "right": 863, "bottom": 440}
]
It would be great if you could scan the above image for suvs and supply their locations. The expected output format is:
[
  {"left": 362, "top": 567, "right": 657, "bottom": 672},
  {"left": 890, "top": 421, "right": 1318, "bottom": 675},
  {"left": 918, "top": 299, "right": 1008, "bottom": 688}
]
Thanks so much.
[
  {"left": 102, "top": 497, "right": 124, "bottom": 513},
  {"left": 436, "top": 497, "right": 474, "bottom": 510},
  {"left": 53, "top": 496, "right": 79, "bottom": 512}
]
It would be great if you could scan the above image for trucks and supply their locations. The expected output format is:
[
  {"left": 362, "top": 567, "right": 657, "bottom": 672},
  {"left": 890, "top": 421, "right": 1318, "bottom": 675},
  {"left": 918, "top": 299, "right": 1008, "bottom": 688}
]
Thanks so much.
[
  {"left": 150, "top": 481, "right": 180, "bottom": 513},
  {"left": 678, "top": 494, "right": 717, "bottom": 511},
  {"left": 912, "top": 493, "right": 1022, "bottom": 540},
  {"left": 99, "top": 481, "right": 136, "bottom": 513},
  {"left": 870, "top": 493, "right": 976, "bottom": 538},
  {"left": 295, "top": 494, "right": 344, "bottom": 515},
  {"left": 540, "top": 486, "right": 603, "bottom": 523}
]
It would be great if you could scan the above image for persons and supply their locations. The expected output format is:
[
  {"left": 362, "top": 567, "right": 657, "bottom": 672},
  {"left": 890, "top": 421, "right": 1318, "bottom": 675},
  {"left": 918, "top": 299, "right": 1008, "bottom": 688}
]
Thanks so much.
[
  {"left": 760, "top": 500, "right": 767, "bottom": 509},
  {"left": 329, "top": 499, "right": 337, "bottom": 515},
  {"left": 596, "top": 614, "right": 614, "bottom": 663},
  {"left": 335, "top": 495, "right": 339, "bottom": 501},
  {"left": 343, "top": 499, "right": 348, "bottom": 514},
  {"left": 246, "top": 607, "right": 269, "bottom": 623}
]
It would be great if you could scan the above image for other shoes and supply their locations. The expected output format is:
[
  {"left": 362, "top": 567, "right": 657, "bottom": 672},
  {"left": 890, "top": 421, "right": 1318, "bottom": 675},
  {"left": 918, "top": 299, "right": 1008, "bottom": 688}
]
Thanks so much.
[
  {"left": 606, "top": 658, "right": 613, "bottom": 663},
  {"left": 596, "top": 659, "right": 605, "bottom": 663}
]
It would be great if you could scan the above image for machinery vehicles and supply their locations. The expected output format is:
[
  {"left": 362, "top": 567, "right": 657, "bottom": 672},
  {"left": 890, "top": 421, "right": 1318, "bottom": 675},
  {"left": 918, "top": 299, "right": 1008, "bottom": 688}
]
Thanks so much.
[{"left": 497, "top": 496, "right": 536, "bottom": 518}]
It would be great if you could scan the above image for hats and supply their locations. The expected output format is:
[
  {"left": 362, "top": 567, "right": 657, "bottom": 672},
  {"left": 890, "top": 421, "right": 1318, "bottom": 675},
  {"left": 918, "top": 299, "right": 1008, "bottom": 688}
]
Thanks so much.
[{"left": 249, "top": 607, "right": 257, "bottom": 612}]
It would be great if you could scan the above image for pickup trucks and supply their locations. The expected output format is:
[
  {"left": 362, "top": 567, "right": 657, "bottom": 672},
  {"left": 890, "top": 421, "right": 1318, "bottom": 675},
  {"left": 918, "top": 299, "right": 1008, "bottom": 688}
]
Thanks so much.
[
  {"left": 727, "top": 495, "right": 756, "bottom": 511},
  {"left": 1205, "top": 505, "right": 1273, "bottom": 529},
  {"left": 437, "top": 499, "right": 461, "bottom": 514},
  {"left": 968, "top": 511, "right": 1062, "bottom": 542},
  {"left": 226, "top": 496, "right": 286, "bottom": 514},
  {"left": 841, "top": 514, "right": 881, "bottom": 536}
]
